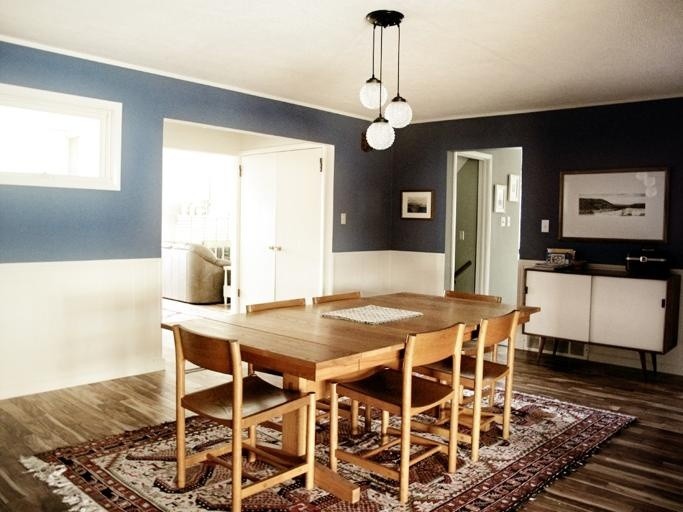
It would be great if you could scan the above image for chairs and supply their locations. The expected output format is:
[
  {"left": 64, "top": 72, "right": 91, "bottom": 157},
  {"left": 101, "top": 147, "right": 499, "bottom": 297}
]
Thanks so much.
[
  {"left": 170, "top": 324, "right": 317, "bottom": 511},
  {"left": 436, "top": 292, "right": 503, "bottom": 416},
  {"left": 412, "top": 309, "right": 520, "bottom": 465},
  {"left": 311, "top": 291, "right": 372, "bottom": 433},
  {"left": 327, "top": 321, "right": 466, "bottom": 506},
  {"left": 245, "top": 298, "right": 352, "bottom": 444}
]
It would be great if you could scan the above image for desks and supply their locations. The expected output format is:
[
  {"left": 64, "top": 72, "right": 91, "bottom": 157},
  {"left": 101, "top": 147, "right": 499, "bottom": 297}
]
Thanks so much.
[{"left": 161, "top": 291, "right": 541, "bottom": 504}]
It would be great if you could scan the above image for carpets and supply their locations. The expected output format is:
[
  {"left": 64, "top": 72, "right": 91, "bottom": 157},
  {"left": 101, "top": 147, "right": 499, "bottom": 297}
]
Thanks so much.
[{"left": 17, "top": 386, "right": 639, "bottom": 511}]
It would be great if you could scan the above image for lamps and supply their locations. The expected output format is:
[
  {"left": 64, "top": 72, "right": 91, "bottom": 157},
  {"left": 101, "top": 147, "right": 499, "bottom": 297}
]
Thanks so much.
[{"left": 358, "top": 10, "right": 412, "bottom": 151}]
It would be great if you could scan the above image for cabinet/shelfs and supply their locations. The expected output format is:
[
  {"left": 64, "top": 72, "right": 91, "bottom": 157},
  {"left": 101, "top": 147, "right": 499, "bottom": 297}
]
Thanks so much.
[
  {"left": 521, "top": 262, "right": 682, "bottom": 377},
  {"left": 236, "top": 146, "right": 325, "bottom": 317}
]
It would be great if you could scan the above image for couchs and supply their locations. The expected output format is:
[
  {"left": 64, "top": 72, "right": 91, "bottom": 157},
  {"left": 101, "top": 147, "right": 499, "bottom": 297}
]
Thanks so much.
[{"left": 162, "top": 241, "right": 229, "bottom": 304}]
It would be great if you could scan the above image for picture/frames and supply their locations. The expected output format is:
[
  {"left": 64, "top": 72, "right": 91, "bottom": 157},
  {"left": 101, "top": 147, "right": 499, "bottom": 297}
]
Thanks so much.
[
  {"left": 494, "top": 184, "right": 506, "bottom": 214},
  {"left": 507, "top": 174, "right": 520, "bottom": 203},
  {"left": 402, "top": 190, "right": 434, "bottom": 220},
  {"left": 559, "top": 170, "right": 666, "bottom": 241}
]
